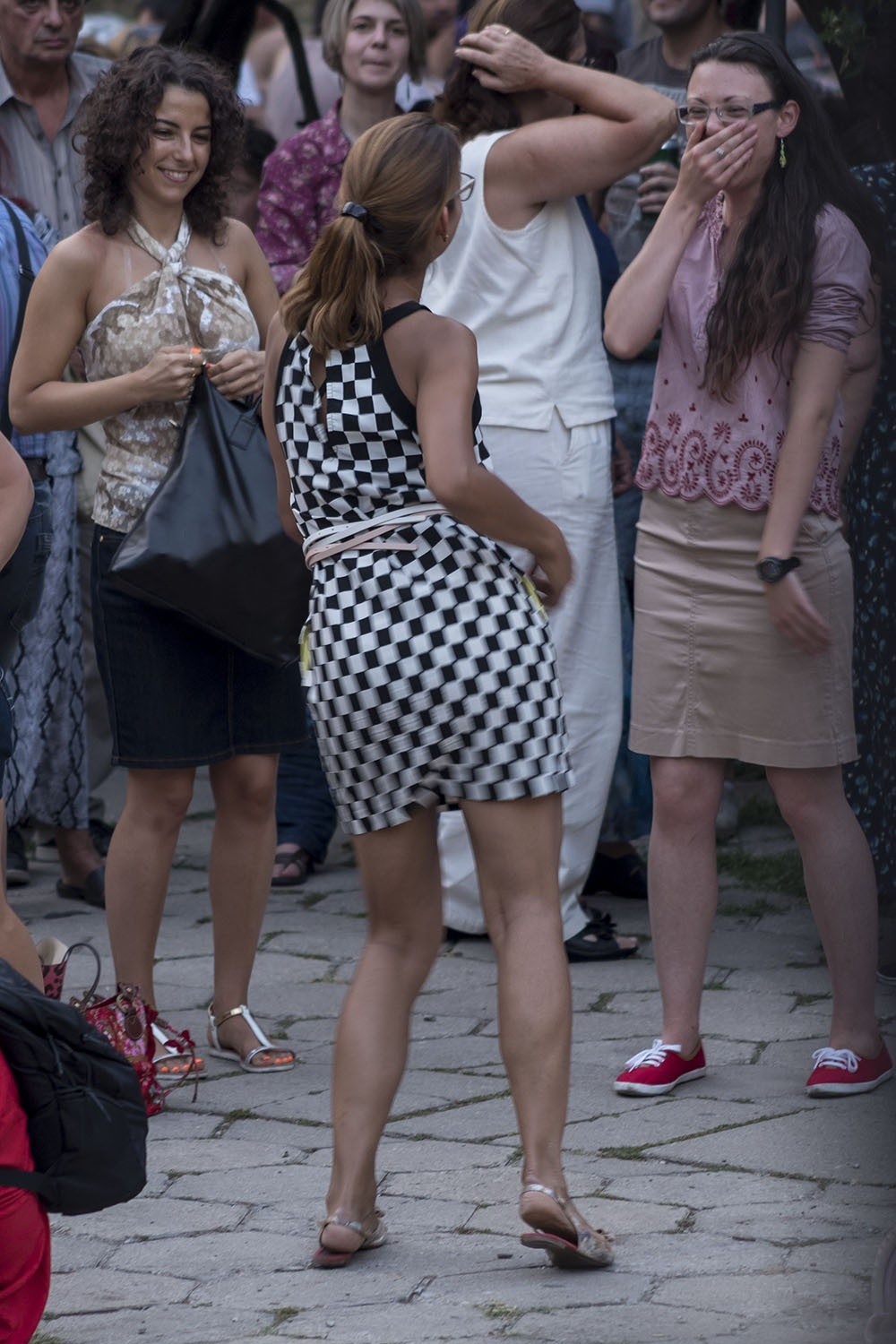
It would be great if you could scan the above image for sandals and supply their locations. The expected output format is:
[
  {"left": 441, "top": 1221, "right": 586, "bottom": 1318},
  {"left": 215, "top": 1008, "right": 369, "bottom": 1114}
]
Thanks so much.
[
  {"left": 151, "top": 1021, "right": 208, "bottom": 1080},
  {"left": 270, "top": 842, "right": 310, "bottom": 886},
  {"left": 207, "top": 999, "right": 297, "bottom": 1074},
  {"left": 308, "top": 1205, "right": 391, "bottom": 1267},
  {"left": 517, "top": 1182, "right": 616, "bottom": 1267},
  {"left": 563, "top": 906, "right": 640, "bottom": 962}
]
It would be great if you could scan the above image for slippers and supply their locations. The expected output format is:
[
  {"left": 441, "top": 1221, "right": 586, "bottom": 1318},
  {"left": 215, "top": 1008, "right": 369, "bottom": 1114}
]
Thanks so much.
[{"left": 56, "top": 866, "right": 106, "bottom": 908}]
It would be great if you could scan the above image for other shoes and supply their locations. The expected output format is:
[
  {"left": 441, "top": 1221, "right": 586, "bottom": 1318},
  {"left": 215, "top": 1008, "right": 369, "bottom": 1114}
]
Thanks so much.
[
  {"left": 89, "top": 818, "right": 115, "bottom": 863},
  {"left": 5, "top": 831, "right": 32, "bottom": 883},
  {"left": 583, "top": 844, "right": 649, "bottom": 897}
]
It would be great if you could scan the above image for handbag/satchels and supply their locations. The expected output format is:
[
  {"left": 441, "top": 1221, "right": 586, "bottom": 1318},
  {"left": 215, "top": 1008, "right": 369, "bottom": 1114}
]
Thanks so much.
[
  {"left": 108, "top": 365, "right": 311, "bottom": 660},
  {"left": 33, "top": 934, "right": 102, "bottom": 1009},
  {"left": 65, "top": 980, "right": 166, "bottom": 1118}
]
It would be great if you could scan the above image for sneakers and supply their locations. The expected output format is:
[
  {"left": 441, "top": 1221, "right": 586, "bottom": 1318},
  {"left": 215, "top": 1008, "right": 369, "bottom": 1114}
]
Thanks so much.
[
  {"left": 805, "top": 1040, "right": 893, "bottom": 1097},
  {"left": 613, "top": 1036, "right": 708, "bottom": 1095}
]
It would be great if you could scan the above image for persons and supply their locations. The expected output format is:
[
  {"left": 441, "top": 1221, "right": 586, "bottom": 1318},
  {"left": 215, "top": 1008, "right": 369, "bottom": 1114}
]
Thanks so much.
[
  {"left": 12, "top": 48, "right": 304, "bottom": 1082},
  {"left": 269, "top": 110, "right": 615, "bottom": 1278},
  {"left": 599, "top": 32, "right": 895, "bottom": 1101},
  {"left": 0, "top": 1, "right": 896, "bottom": 1344}
]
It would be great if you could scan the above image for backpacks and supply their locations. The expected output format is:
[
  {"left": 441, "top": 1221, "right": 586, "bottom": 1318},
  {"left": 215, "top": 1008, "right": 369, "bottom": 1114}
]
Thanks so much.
[{"left": 0, "top": 958, "right": 152, "bottom": 1215}]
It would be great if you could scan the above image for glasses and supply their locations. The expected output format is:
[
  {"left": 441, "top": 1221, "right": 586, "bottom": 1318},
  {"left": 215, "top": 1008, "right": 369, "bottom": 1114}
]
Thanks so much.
[
  {"left": 444, "top": 171, "right": 476, "bottom": 204},
  {"left": 675, "top": 97, "right": 781, "bottom": 127}
]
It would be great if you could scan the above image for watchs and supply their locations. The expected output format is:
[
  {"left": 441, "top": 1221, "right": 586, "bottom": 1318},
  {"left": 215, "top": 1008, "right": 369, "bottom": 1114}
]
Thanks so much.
[{"left": 755, "top": 555, "right": 802, "bottom": 585}]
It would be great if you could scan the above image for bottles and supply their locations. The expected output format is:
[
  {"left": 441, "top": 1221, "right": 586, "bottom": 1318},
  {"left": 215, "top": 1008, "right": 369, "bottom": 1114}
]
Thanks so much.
[{"left": 630, "top": 133, "right": 681, "bottom": 229}]
